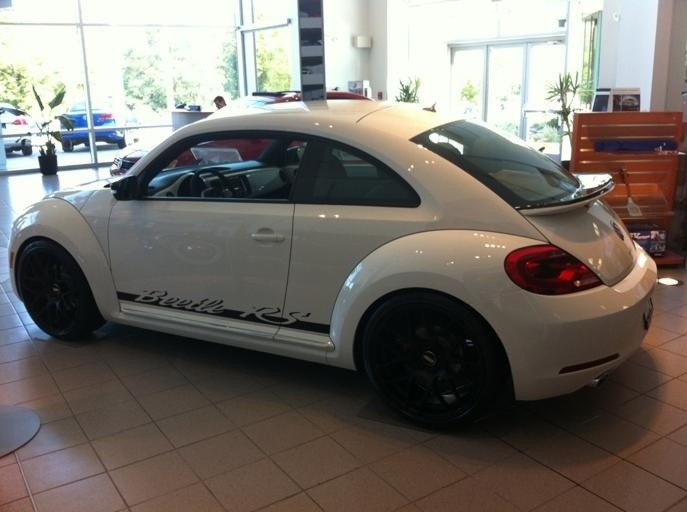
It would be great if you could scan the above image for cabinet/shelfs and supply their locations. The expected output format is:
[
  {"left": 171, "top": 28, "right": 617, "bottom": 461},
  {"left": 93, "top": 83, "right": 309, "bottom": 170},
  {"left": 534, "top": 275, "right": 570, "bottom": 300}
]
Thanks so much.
[{"left": 568, "top": 110, "right": 685, "bottom": 268}]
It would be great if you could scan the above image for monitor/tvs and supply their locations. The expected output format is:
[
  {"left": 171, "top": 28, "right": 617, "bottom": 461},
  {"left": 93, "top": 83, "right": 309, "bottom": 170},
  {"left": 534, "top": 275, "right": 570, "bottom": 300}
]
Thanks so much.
[
  {"left": 188, "top": 106, "right": 200, "bottom": 111},
  {"left": 591, "top": 87, "right": 610, "bottom": 112}
]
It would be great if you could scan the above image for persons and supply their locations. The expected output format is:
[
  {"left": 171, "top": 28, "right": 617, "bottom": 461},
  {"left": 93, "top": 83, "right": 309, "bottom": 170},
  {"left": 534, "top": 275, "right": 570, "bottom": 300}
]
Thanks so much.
[{"left": 214, "top": 96, "right": 225, "bottom": 109}]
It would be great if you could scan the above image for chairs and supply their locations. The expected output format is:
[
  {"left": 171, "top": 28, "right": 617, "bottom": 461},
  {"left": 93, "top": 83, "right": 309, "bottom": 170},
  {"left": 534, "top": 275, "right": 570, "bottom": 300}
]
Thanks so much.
[{"left": 310, "top": 141, "right": 461, "bottom": 201}]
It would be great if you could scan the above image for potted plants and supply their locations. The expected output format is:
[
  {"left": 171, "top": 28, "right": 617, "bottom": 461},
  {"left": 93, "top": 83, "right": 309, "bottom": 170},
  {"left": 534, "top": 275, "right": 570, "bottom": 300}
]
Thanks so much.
[{"left": 21, "top": 84, "right": 67, "bottom": 176}]
